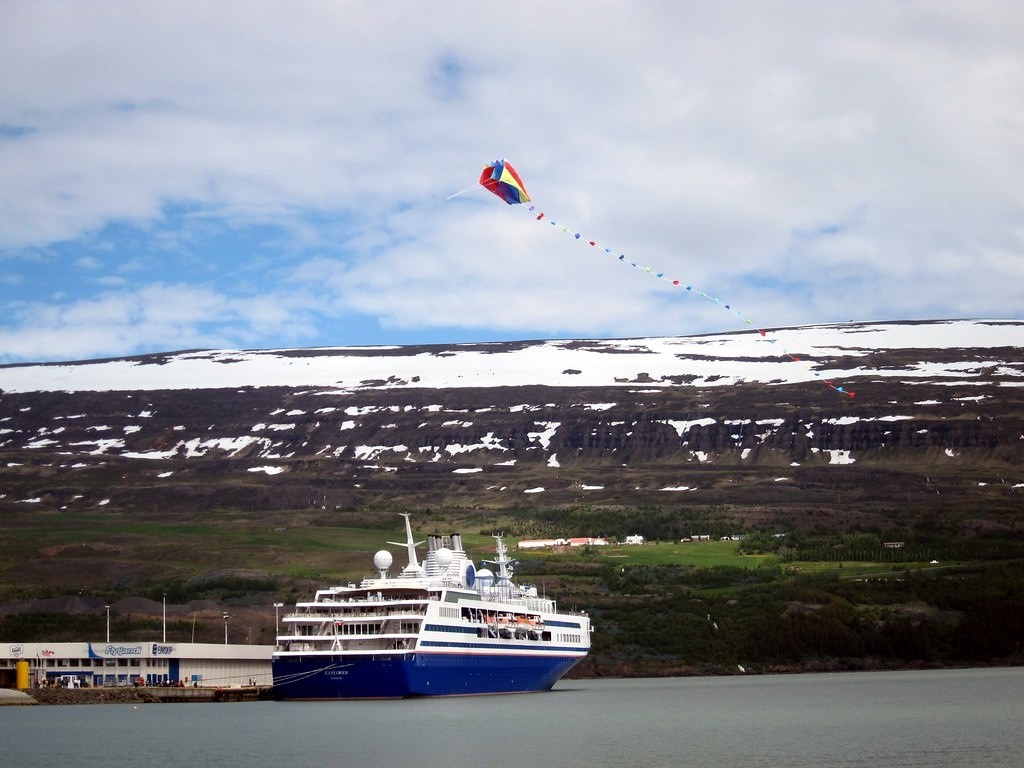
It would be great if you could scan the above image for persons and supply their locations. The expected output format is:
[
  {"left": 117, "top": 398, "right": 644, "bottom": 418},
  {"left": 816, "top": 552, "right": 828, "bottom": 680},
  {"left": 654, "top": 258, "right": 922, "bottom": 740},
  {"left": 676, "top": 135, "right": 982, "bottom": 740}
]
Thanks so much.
[
  {"left": 248, "top": 677, "right": 257, "bottom": 687},
  {"left": 40, "top": 675, "right": 87, "bottom": 688},
  {"left": 133, "top": 677, "right": 199, "bottom": 687}
]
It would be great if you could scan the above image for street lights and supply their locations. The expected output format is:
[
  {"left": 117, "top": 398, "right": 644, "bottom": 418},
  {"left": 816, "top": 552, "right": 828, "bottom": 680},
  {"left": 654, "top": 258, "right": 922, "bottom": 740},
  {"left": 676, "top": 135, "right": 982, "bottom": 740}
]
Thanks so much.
[
  {"left": 273, "top": 602, "right": 283, "bottom": 646},
  {"left": 105, "top": 605, "right": 110, "bottom": 643},
  {"left": 222, "top": 612, "right": 229, "bottom": 644},
  {"left": 162, "top": 593, "right": 168, "bottom": 642}
]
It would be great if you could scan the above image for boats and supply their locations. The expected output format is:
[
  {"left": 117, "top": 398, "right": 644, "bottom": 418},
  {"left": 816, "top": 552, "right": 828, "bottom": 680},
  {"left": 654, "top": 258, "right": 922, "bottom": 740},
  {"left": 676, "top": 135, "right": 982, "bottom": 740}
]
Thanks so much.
[{"left": 271, "top": 510, "right": 594, "bottom": 701}]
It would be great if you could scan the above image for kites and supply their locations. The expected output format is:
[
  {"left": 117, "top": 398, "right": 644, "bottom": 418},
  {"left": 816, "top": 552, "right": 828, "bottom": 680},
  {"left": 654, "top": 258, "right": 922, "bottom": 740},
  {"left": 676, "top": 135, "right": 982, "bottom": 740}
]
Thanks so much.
[{"left": 479, "top": 159, "right": 531, "bottom": 205}]
